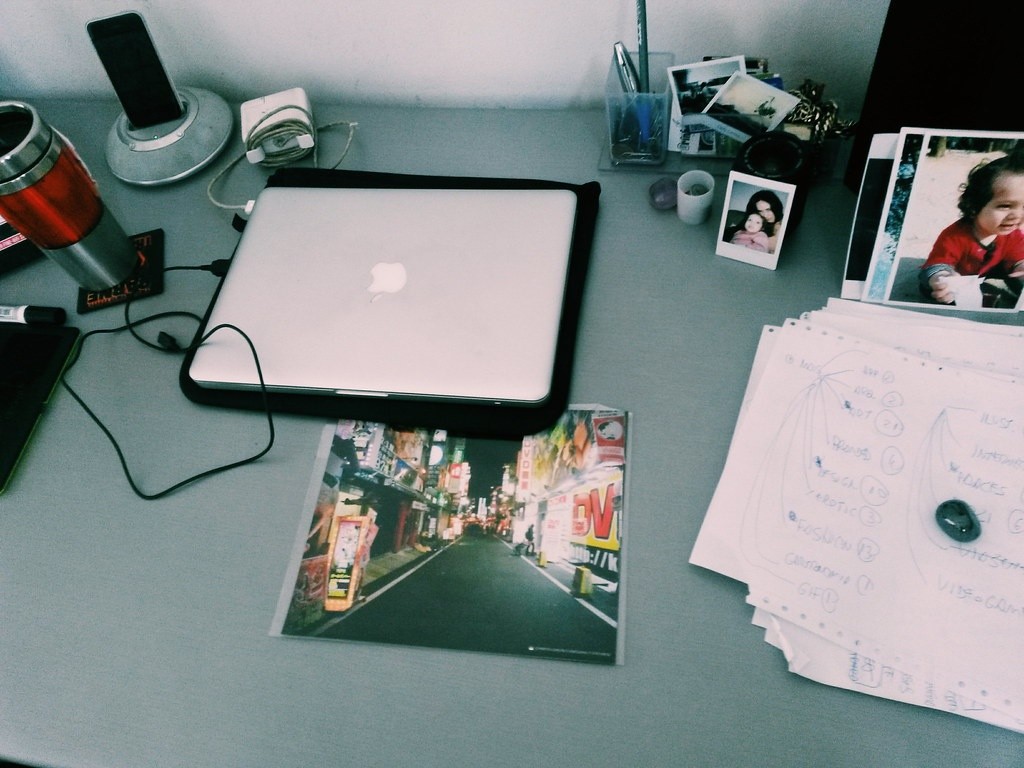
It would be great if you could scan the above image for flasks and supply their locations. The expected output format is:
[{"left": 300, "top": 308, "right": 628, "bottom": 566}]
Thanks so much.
[{"left": 0, "top": 101, "right": 136, "bottom": 290}]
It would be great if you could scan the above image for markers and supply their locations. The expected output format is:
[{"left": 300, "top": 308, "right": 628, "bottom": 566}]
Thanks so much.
[{"left": 0, "top": 305, "right": 66, "bottom": 326}]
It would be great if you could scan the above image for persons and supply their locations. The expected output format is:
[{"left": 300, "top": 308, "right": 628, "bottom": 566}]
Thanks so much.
[
  {"left": 918, "top": 151, "right": 1024, "bottom": 306},
  {"left": 526, "top": 525, "right": 536, "bottom": 556},
  {"left": 730, "top": 190, "right": 783, "bottom": 254},
  {"left": 700, "top": 82, "right": 709, "bottom": 104}
]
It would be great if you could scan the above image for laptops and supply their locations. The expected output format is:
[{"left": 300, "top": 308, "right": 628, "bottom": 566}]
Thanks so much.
[{"left": 189, "top": 189, "right": 578, "bottom": 407}]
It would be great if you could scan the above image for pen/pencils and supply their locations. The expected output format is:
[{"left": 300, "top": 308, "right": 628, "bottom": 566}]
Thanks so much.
[{"left": 614, "top": 1, "right": 650, "bottom": 146}]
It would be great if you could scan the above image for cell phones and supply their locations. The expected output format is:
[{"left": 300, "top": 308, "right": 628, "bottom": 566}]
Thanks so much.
[{"left": 85, "top": 10, "right": 186, "bottom": 129}]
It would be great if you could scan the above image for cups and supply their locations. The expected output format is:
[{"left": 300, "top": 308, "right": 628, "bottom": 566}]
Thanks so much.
[{"left": 676, "top": 170, "right": 715, "bottom": 224}]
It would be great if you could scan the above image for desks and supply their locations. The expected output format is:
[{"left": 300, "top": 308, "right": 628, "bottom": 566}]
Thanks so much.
[{"left": 0, "top": 93, "right": 1024, "bottom": 768}]
